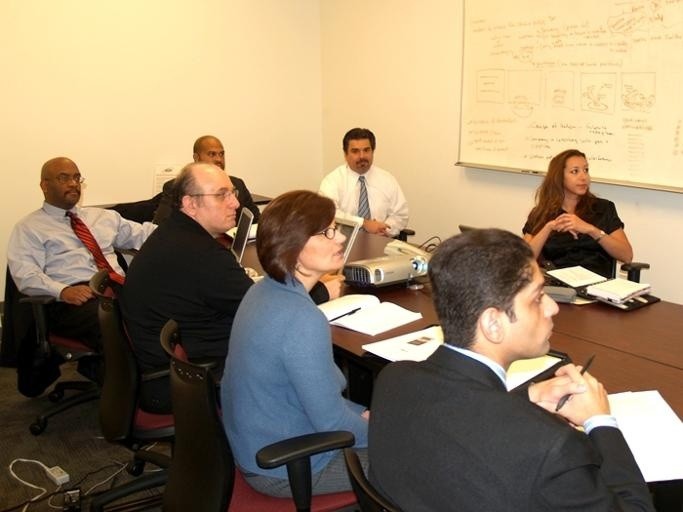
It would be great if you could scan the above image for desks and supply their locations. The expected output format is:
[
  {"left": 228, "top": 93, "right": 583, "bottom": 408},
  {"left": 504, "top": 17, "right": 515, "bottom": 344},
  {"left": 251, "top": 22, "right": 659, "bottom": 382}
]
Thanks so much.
[{"left": 152, "top": 190, "right": 683, "bottom": 509}]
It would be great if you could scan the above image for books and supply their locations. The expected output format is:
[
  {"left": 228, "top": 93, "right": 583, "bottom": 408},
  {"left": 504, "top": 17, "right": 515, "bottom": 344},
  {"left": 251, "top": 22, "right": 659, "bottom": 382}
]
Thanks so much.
[
  {"left": 317, "top": 294, "right": 423, "bottom": 334},
  {"left": 587, "top": 277, "right": 650, "bottom": 304}
]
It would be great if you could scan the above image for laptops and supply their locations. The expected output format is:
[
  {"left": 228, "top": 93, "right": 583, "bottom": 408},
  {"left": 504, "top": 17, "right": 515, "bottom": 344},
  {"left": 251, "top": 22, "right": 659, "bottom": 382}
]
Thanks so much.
[
  {"left": 327, "top": 218, "right": 359, "bottom": 276},
  {"left": 231, "top": 207, "right": 255, "bottom": 263}
]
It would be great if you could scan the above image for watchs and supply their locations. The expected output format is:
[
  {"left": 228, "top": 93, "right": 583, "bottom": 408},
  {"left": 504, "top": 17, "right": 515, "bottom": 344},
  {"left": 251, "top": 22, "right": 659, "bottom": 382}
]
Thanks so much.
[{"left": 595, "top": 230, "right": 606, "bottom": 243}]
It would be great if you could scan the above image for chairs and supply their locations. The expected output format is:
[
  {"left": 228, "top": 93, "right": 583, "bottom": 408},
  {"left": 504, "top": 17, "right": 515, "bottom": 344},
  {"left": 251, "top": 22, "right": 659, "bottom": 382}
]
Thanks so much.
[
  {"left": 341, "top": 443, "right": 406, "bottom": 512},
  {"left": 157, "top": 319, "right": 356, "bottom": 510},
  {"left": 4, "top": 263, "right": 103, "bottom": 436},
  {"left": 87, "top": 269, "right": 175, "bottom": 509},
  {"left": 611, "top": 260, "right": 648, "bottom": 283}
]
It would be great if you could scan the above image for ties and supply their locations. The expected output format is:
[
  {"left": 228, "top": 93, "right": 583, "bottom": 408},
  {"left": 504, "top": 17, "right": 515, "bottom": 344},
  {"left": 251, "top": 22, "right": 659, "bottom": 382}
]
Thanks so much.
[
  {"left": 63, "top": 210, "right": 125, "bottom": 285},
  {"left": 357, "top": 175, "right": 372, "bottom": 221}
]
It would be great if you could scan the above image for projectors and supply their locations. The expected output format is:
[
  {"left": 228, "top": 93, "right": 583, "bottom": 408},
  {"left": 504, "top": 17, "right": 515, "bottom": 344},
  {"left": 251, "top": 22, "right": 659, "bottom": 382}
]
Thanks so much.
[{"left": 341, "top": 255, "right": 428, "bottom": 288}]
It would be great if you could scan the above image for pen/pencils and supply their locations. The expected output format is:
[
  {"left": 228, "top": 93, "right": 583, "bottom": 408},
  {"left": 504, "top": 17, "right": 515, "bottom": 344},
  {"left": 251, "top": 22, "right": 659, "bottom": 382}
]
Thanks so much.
[
  {"left": 328, "top": 308, "right": 360, "bottom": 324},
  {"left": 555, "top": 356, "right": 593, "bottom": 412}
]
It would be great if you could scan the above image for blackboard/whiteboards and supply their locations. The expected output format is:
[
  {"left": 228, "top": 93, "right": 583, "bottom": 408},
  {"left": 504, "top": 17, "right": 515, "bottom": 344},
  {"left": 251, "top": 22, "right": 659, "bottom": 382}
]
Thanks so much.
[{"left": 454, "top": 1, "right": 683, "bottom": 194}]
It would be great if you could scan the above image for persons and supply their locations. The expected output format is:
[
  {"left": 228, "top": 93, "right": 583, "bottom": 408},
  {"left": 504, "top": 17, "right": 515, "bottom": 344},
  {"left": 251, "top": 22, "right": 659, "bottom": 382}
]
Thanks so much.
[
  {"left": 219, "top": 187, "right": 371, "bottom": 497},
  {"left": 521, "top": 148, "right": 633, "bottom": 280},
  {"left": 152, "top": 135, "right": 262, "bottom": 227},
  {"left": 366, "top": 227, "right": 655, "bottom": 512},
  {"left": 7, "top": 157, "right": 159, "bottom": 348},
  {"left": 118, "top": 161, "right": 346, "bottom": 416},
  {"left": 316, "top": 127, "right": 410, "bottom": 239}
]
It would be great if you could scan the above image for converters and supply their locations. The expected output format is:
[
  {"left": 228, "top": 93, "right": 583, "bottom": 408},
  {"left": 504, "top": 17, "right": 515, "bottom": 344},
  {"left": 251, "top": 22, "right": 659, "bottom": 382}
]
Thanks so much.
[{"left": 45, "top": 466, "right": 70, "bottom": 484}]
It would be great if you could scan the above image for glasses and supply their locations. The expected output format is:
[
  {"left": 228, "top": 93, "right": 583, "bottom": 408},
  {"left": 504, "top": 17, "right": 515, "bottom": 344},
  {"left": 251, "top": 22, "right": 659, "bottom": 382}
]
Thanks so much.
[
  {"left": 189, "top": 187, "right": 239, "bottom": 201},
  {"left": 307, "top": 222, "right": 341, "bottom": 239},
  {"left": 42, "top": 174, "right": 86, "bottom": 184}
]
